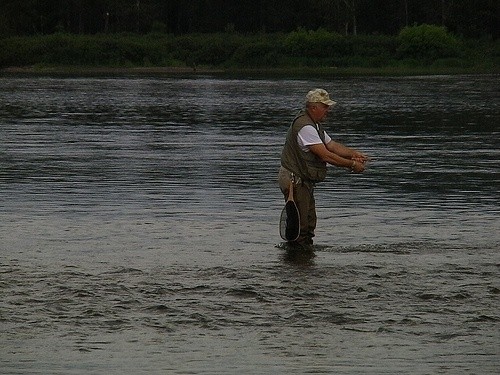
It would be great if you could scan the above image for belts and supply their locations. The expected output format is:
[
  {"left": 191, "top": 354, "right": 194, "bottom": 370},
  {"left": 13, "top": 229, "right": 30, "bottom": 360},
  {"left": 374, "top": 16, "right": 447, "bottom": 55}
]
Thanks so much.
[{"left": 282, "top": 165, "right": 297, "bottom": 175}]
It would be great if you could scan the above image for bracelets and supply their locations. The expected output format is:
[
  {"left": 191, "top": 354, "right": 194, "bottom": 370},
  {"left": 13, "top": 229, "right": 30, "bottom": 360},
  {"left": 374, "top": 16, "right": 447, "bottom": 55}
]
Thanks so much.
[{"left": 351, "top": 160, "right": 356, "bottom": 167}]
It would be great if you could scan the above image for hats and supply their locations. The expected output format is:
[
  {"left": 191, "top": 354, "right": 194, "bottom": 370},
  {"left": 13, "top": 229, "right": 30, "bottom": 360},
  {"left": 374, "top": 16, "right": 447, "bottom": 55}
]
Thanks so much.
[{"left": 306, "top": 88, "right": 336, "bottom": 106}]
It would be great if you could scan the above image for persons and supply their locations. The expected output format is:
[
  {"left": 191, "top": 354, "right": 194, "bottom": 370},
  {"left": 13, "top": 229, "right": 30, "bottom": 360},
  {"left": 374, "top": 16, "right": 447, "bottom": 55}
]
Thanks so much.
[{"left": 278, "top": 88, "right": 368, "bottom": 243}]
